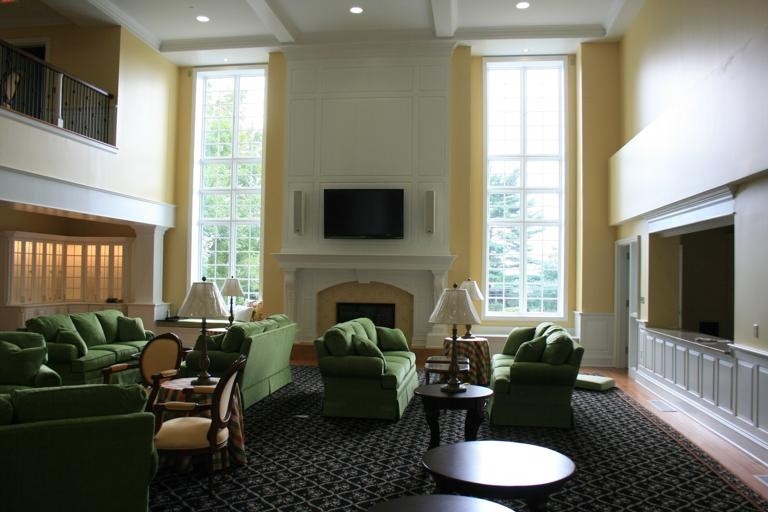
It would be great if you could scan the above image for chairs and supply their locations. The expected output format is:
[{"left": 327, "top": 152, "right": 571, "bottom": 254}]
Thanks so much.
[{"left": 100, "top": 331, "right": 248, "bottom": 501}]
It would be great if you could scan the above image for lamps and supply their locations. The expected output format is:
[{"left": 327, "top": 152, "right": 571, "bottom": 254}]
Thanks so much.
[
  {"left": 431, "top": 278, "right": 484, "bottom": 393},
  {"left": 176, "top": 274, "right": 247, "bottom": 385}
]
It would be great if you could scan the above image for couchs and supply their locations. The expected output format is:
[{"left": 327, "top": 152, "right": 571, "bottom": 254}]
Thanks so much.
[
  {"left": 489, "top": 319, "right": 585, "bottom": 431},
  {"left": 0, "top": 306, "right": 156, "bottom": 511},
  {"left": 313, "top": 315, "right": 419, "bottom": 420},
  {"left": 179, "top": 313, "right": 300, "bottom": 412}
]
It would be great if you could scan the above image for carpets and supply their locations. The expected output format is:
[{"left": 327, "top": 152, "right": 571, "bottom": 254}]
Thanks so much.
[{"left": 150, "top": 363, "right": 767, "bottom": 510}]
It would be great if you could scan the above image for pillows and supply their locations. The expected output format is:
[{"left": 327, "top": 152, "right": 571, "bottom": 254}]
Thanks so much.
[
  {"left": 501, "top": 327, "right": 549, "bottom": 364},
  {"left": 352, "top": 326, "right": 410, "bottom": 370},
  {"left": 234, "top": 299, "right": 265, "bottom": 322}
]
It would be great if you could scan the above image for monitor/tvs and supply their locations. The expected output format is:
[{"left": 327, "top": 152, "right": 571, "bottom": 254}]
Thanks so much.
[{"left": 323, "top": 188, "right": 405, "bottom": 240}]
